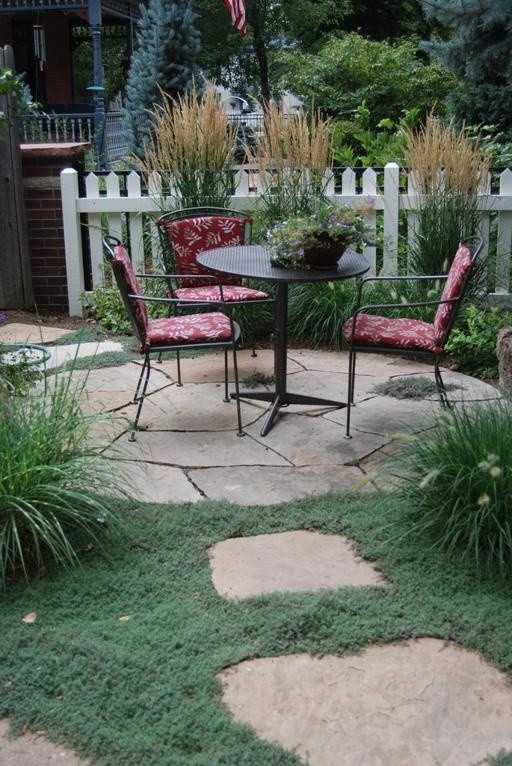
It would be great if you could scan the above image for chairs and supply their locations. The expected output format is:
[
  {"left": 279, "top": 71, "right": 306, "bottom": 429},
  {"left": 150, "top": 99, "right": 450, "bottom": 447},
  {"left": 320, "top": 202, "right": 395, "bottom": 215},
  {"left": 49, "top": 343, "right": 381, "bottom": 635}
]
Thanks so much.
[
  {"left": 342, "top": 235, "right": 484, "bottom": 439},
  {"left": 155, "top": 206, "right": 275, "bottom": 389},
  {"left": 101, "top": 235, "right": 245, "bottom": 442}
]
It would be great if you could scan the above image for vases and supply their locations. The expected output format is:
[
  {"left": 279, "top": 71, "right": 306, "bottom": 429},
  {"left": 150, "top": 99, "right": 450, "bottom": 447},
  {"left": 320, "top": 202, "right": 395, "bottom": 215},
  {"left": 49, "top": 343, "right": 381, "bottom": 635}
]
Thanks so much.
[{"left": 285, "top": 229, "right": 354, "bottom": 269}]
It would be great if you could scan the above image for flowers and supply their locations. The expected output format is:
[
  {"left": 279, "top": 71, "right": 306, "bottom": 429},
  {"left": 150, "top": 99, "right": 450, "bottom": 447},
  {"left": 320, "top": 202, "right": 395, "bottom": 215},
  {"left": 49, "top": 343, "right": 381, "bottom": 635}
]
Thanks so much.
[{"left": 267, "top": 196, "right": 371, "bottom": 273}]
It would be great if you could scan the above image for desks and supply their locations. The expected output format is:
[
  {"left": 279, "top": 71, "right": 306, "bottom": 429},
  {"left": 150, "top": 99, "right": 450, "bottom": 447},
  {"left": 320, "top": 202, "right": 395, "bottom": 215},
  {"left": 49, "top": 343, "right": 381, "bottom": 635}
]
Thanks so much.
[{"left": 197, "top": 244, "right": 369, "bottom": 436}]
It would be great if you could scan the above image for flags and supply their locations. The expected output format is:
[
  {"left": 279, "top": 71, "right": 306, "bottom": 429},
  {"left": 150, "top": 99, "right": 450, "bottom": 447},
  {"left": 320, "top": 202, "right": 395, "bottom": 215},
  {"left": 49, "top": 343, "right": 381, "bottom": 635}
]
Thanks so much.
[{"left": 223, "top": 0, "right": 248, "bottom": 34}]
